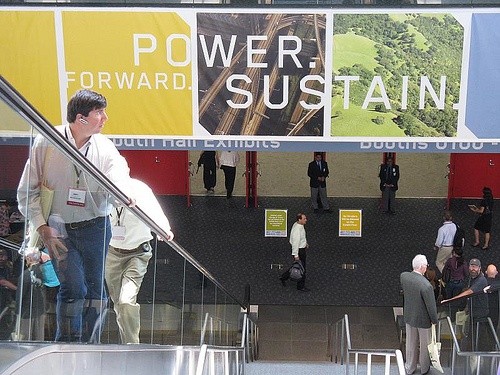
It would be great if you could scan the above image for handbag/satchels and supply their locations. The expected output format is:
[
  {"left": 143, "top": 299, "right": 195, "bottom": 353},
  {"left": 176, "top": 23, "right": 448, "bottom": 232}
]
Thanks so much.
[
  {"left": 427, "top": 321, "right": 444, "bottom": 374},
  {"left": 478, "top": 199, "right": 490, "bottom": 217}
]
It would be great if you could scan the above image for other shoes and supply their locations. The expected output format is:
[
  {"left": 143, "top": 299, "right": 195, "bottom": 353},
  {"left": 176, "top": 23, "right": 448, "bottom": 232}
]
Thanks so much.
[
  {"left": 327, "top": 208, "right": 333, "bottom": 212},
  {"left": 383, "top": 210, "right": 397, "bottom": 214},
  {"left": 297, "top": 288, "right": 311, "bottom": 292},
  {"left": 206, "top": 189, "right": 214, "bottom": 194},
  {"left": 421, "top": 365, "right": 430, "bottom": 375},
  {"left": 315, "top": 209, "right": 318, "bottom": 213},
  {"left": 472, "top": 243, "right": 480, "bottom": 247},
  {"left": 481, "top": 245, "right": 489, "bottom": 250},
  {"left": 279, "top": 275, "right": 287, "bottom": 287},
  {"left": 407, "top": 364, "right": 417, "bottom": 375}
]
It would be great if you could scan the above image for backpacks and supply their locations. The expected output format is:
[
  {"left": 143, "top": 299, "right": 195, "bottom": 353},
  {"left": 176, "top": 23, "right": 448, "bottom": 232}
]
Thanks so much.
[{"left": 288, "top": 260, "right": 305, "bottom": 282}]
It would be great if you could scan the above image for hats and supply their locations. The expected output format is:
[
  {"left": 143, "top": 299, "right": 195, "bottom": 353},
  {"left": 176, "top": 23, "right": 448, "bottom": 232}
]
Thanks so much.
[{"left": 469, "top": 258, "right": 481, "bottom": 267}]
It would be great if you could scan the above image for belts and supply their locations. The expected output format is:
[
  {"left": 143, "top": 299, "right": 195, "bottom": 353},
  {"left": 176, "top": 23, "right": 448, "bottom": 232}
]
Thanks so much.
[
  {"left": 442, "top": 245, "right": 454, "bottom": 247},
  {"left": 64, "top": 217, "right": 102, "bottom": 231},
  {"left": 114, "top": 248, "right": 141, "bottom": 253}
]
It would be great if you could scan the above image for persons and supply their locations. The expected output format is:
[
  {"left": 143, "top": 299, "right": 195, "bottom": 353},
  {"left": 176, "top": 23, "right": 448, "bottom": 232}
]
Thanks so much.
[
  {"left": 308, "top": 152, "right": 335, "bottom": 214},
  {"left": 433, "top": 210, "right": 465, "bottom": 273},
  {"left": 0, "top": 197, "right": 61, "bottom": 344},
  {"left": 104, "top": 155, "right": 174, "bottom": 343},
  {"left": 424, "top": 247, "right": 500, "bottom": 375},
  {"left": 400, "top": 254, "right": 438, "bottom": 375},
  {"left": 17, "top": 90, "right": 137, "bottom": 343},
  {"left": 377, "top": 158, "right": 400, "bottom": 216},
  {"left": 196, "top": 151, "right": 219, "bottom": 193},
  {"left": 469, "top": 187, "right": 494, "bottom": 251},
  {"left": 279, "top": 212, "right": 312, "bottom": 292},
  {"left": 220, "top": 150, "right": 240, "bottom": 199}
]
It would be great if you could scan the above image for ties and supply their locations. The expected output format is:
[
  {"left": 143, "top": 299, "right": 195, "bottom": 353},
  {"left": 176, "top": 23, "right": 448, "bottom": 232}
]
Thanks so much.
[{"left": 317, "top": 161, "right": 320, "bottom": 171}]
